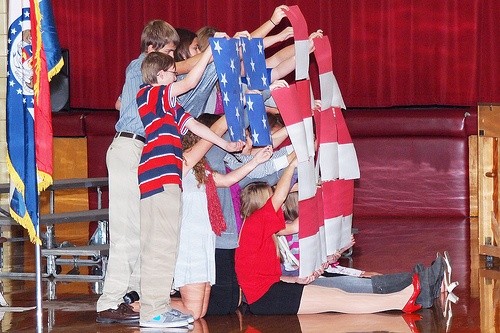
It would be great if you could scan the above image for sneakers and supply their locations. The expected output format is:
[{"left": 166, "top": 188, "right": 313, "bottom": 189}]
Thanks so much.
[
  {"left": 138, "top": 313, "right": 189, "bottom": 328},
  {"left": 169, "top": 307, "right": 195, "bottom": 324},
  {"left": 141, "top": 324, "right": 195, "bottom": 333}
]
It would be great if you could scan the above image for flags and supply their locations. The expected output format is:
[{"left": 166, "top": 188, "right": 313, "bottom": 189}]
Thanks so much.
[{"left": 6, "top": 0, "right": 65, "bottom": 245}]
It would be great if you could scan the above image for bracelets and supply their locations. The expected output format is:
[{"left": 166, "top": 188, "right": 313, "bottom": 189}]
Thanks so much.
[
  {"left": 295, "top": 276, "right": 298, "bottom": 283},
  {"left": 270, "top": 19, "right": 275, "bottom": 26}
]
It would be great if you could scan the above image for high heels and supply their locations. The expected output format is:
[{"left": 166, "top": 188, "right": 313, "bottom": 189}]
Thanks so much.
[
  {"left": 402, "top": 271, "right": 423, "bottom": 314},
  {"left": 442, "top": 292, "right": 460, "bottom": 317},
  {"left": 402, "top": 312, "right": 424, "bottom": 333},
  {"left": 436, "top": 250, "right": 459, "bottom": 294}
]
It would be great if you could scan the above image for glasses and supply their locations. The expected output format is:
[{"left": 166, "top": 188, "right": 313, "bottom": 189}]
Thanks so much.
[{"left": 157, "top": 69, "right": 178, "bottom": 77}]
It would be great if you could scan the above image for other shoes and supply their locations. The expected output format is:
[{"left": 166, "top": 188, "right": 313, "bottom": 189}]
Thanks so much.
[
  {"left": 170, "top": 289, "right": 183, "bottom": 299},
  {"left": 124, "top": 291, "right": 140, "bottom": 304}
]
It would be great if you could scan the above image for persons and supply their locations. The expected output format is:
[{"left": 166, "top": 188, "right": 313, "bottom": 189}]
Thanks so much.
[{"left": 95, "top": 5, "right": 460, "bottom": 326}]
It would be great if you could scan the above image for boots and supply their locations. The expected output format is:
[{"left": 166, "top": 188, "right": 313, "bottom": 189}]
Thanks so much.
[{"left": 370, "top": 256, "right": 444, "bottom": 309}]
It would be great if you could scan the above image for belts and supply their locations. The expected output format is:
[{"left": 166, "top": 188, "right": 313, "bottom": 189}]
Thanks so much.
[{"left": 115, "top": 132, "right": 145, "bottom": 143}]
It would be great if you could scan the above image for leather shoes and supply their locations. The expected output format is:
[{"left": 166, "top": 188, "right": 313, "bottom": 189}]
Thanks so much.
[{"left": 95, "top": 302, "right": 140, "bottom": 324}]
